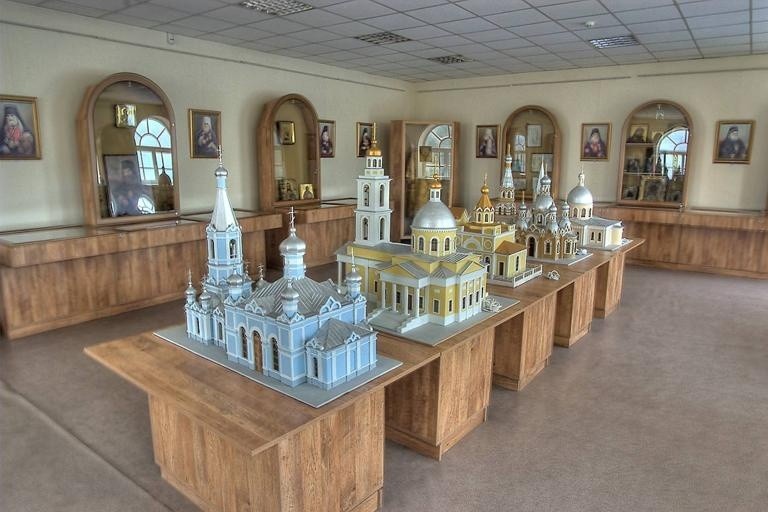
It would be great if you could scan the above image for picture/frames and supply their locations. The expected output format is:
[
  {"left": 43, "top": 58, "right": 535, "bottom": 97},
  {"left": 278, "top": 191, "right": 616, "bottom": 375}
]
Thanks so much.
[
  {"left": 355, "top": 119, "right": 377, "bottom": 160},
  {"left": 275, "top": 119, "right": 296, "bottom": 148},
  {"left": 577, "top": 119, "right": 610, "bottom": 162},
  {"left": 100, "top": 150, "right": 146, "bottom": 220},
  {"left": 509, "top": 120, "right": 554, "bottom": 195},
  {"left": 0, "top": 92, "right": 43, "bottom": 164},
  {"left": 621, "top": 117, "right": 684, "bottom": 204},
  {"left": 317, "top": 116, "right": 338, "bottom": 159},
  {"left": 710, "top": 117, "right": 756, "bottom": 165},
  {"left": 112, "top": 102, "right": 138, "bottom": 130},
  {"left": 473, "top": 124, "right": 501, "bottom": 159},
  {"left": 186, "top": 107, "right": 223, "bottom": 161}
]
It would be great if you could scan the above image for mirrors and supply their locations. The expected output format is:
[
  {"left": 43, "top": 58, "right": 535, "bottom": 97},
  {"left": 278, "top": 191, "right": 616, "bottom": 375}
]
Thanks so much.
[
  {"left": 254, "top": 91, "right": 326, "bottom": 214},
  {"left": 68, "top": 69, "right": 185, "bottom": 232},
  {"left": 614, "top": 97, "right": 696, "bottom": 210},
  {"left": 497, "top": 100, "right": 563, "bottom": 203},
  {"left": 386, "top": 118, "right": 463, "bottom": 244}
]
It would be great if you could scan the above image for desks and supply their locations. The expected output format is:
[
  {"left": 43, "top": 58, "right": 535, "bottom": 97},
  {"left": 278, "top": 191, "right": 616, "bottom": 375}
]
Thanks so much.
[
  {"left": 357, "top": 289, "right": 536, "bottom": 466},
  {"left": 487, "top": 195, "right": 768, "bottom": 282},
  {"left": 576, "top": 235, "right": 646, "bottom": 319},
  {"left": 525, "top": 246, "right": 621, "bottom": 349},
  {"left": 82, "top": 303, "right": 442, "bottom": 512},
  {"left": 482, "top": 257, "right": 589, "bottom": 392},
  {"left": 251, "top": 196, "right": 396, "bottom": 275},
  {"left": 0, "top": 203, "right": 287, "bottom": 343}
]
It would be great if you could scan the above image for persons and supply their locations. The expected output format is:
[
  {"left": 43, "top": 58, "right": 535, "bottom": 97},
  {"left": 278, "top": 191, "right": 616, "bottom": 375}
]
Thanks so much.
[
  {"left": 322, "top": 125, "right": 332, "bottom": 155},
  {"left": 585, "top": 127, "right": 606, "bottom": 156},
  {"left": 1, "top": 105, "right": 34, "bottom": 156},
  {"left": 721, "top": 124, "right": 750, "bottom": 160},
  {"left": 195, "top": 116, "right": 218, "bottom": 156},
  {"left": 111, "top": 159, "right": 142, "bottom": 212}
]
